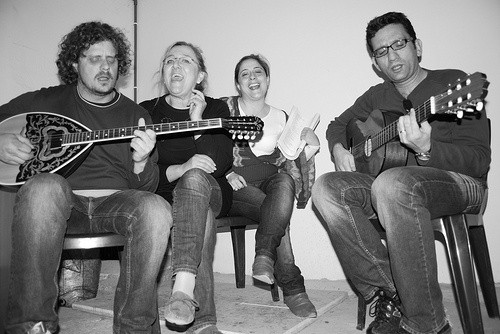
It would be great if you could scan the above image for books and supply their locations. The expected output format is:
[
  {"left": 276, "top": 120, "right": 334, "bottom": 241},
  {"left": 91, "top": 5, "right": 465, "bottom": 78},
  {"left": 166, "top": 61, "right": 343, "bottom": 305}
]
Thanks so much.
[{"left": 278, "top": 106, "right": 320, "bottom": 160}]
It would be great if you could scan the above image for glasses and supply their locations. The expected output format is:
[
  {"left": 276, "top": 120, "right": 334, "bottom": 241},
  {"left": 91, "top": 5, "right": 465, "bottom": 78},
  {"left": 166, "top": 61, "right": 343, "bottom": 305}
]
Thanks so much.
[
  {"left": 163, "top": 57, "right": 200, "bottom": 67},
  {"left": 372, "top": 38, "right": 413, "bottom": 58}
]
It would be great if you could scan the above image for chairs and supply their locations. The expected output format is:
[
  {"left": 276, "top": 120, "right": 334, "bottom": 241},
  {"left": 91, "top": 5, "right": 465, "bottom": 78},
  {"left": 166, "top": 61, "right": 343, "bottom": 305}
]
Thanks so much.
[
  {"left": 64, "top": 233, "right": 126, "bottom": 250},
  {"left": 215, "top": 214, "right": 280, "bottom": 302},
  {"left": 355, "top": 118, "right": 500, "bottom": 334}
]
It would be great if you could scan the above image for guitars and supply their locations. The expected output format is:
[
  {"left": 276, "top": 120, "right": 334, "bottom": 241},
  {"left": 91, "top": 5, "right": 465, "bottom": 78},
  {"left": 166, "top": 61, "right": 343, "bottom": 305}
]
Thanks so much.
[
  {"left": 346, "top": 71, "right": 490, "bottom": 177},
  {"left": 0, "top": 111, "right": 264, "bottom": 191}
]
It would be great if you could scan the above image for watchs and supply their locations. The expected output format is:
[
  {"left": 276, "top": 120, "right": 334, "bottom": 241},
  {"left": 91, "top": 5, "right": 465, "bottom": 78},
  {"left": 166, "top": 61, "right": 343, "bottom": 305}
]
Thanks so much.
[{"left": 416, "top": 150, "right": 431, "bottom": 160}]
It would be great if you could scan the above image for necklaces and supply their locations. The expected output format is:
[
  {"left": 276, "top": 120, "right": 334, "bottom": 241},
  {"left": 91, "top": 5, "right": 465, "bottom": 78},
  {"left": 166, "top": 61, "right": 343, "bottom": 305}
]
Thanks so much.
[{"left": 77, "top": 85, "right": 121, "bottom": 107}]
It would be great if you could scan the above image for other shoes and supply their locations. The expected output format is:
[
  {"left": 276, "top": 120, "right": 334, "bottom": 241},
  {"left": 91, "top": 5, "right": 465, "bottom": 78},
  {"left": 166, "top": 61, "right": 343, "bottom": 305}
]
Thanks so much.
[
  {"left": 199, "top": 325, "right": 223, "bottom": 334},
  {"left": 252, "top": 254, "right": 275, "bottom": 285},
  {"left": 164, "top": 289, "right": 200, "bottom": 326},
  {"left": 283, "top": 292, "right": 317, "bottom": 318}
]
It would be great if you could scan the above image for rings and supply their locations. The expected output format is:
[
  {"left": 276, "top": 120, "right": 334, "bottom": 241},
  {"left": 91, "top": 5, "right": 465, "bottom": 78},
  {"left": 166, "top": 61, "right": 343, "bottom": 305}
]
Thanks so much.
[{"left": 400, "top": 129, "right": 406, "bottom": 133}]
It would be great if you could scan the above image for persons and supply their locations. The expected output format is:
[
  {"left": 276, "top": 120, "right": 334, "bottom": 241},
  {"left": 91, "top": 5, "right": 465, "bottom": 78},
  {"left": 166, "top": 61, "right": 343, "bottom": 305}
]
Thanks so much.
[
  {"left": 138, "top": 42, "right": 233, "bottom": 334},
  {"left": 0, "top": 21, "right": 172, "bottom": 334},
  {"left": 218, "top": 55, "right": 321, "bottom": 318},
  {"left": 311, "top": 11, "right": 491, "bottom": 334}
]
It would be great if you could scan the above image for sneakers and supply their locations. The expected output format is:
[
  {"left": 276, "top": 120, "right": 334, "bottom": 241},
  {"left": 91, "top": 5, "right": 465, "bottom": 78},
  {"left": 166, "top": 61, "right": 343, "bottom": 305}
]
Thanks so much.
[{"left": 366, "top": 290, "right": 402, "bottom": 334}]
